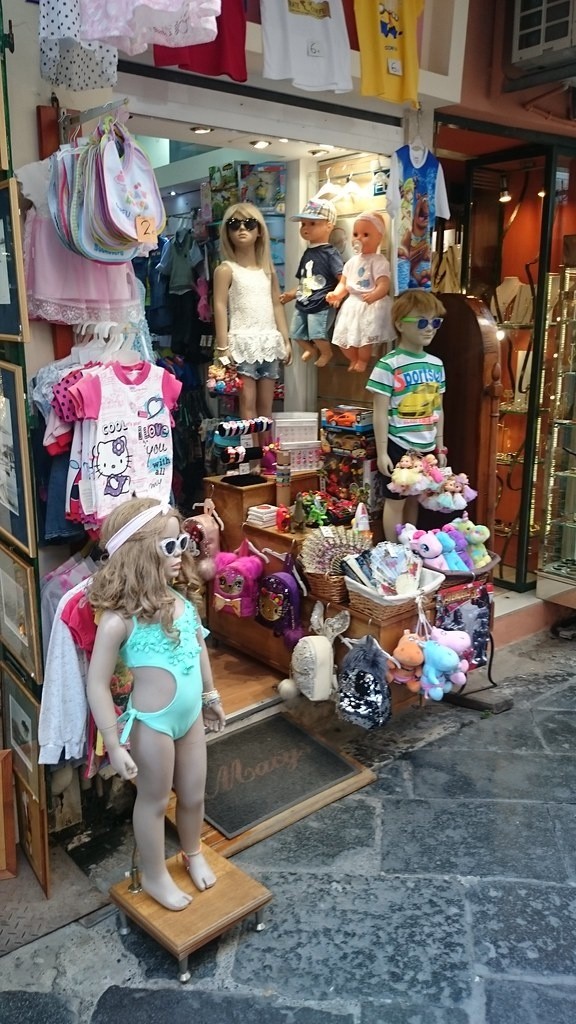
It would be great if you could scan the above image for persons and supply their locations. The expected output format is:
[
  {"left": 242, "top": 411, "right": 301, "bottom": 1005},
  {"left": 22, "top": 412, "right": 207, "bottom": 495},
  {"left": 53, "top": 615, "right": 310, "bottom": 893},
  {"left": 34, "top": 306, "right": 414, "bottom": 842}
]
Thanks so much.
[
  {"left": 387, "top": 448, "right": 479, "bottom": 513},
  {"left": 85, "top": 498, "right": 227, "bottom": 911},
  {"left": 279, "top": 198, "right": 344, "bottom": 366},
  {"left": 365, "top": 287, "right": 449, "bottom": 546},
  {"left": 326, "top": 211, "right": 399, "bottom": 373},
  {"left": 213, "top": 203, "right": 293, "bottom": 470}
]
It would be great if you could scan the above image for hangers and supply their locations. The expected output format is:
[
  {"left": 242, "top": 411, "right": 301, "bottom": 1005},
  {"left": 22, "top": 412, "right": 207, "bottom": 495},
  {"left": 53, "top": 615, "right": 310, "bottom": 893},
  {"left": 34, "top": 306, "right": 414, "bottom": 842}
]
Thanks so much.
[
  {"left": 360, "top": 158, "right": 389, "bottom": 198},
  {"left": 330, "top": 164, "right": 369, "bottom": 202},
  {"left": 87, "top": 542, "right": 102, "bottom": 562},
  {"left": 314, "top": 167, "right": 354, "bottom": 206},
  {"left": 74, "top": 540, "right": 93, "bottom": 562},
  {"left": 57, "top": 101, "right": 119, "bottom": 147},
  {"left": 411, "top": 108, "right": 425, "bottom": 151},
  {"left": 176, "top": 214, "right": 192, "bottom": 239},
  {"left": 56, "top": 320, "right": 142, "bottom": 366}
]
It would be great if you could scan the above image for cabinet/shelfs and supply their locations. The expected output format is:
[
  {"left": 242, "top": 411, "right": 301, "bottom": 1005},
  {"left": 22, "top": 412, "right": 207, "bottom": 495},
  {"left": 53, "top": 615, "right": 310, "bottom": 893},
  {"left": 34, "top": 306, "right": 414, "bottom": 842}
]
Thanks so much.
[
  {"left": 493, "top": 323, "right": 539, "bottom": 593},
  {"left": 203, "top": 473, "right": 437, "bottom": 711},
  {"left": 533, "top": 264, "right": 576, "bottom": 609}
]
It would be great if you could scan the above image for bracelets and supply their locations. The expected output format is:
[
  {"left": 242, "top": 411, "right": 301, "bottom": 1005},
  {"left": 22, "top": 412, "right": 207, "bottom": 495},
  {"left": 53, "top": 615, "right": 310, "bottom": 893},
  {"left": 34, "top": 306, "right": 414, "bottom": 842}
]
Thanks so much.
[
  {"left": 435, "top": 447, "right": 448, "bottom": 453},
  {"left": 202, "top": 690, "right": 220, "bottom": 706},
  {"left": 217, "top": 345, "right": 229, "bottom": 352}
]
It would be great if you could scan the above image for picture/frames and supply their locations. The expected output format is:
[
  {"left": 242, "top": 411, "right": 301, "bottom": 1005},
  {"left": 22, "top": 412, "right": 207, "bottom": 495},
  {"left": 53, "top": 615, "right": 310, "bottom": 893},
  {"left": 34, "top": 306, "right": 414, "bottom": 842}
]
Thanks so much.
[
  {"left": 0, "top": 361, "right": 37, "bottom": 559},
  {"left": 0, "top": 545, "right": 44, "bottom": 686},
  {"left": 0, "top": 660, "right": 45, "bottom": 811},
  {"left": 0, "top": 178, "right": 30, "bottom": 344},
  {"left": 16, "top": 777, "right": 46, "bottom": 887}
]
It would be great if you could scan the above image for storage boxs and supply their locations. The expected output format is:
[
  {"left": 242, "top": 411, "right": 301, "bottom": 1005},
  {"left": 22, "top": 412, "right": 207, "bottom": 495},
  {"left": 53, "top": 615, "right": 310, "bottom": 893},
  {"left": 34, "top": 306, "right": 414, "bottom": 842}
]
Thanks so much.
[
  {"left": 209, "top": 160, "right": 249, "bottom": 222},
  {"left": 240, "top": 162, "right": 287, "bottom": 216},
  {"left": 321, "top": 407, "right": 377, "bottom": 460},
  {"left": 200, "top": 182, "right": 212, "bottom": 222},
  {"left": 320, "top": 454, "right": 385, "bottom": 514}
]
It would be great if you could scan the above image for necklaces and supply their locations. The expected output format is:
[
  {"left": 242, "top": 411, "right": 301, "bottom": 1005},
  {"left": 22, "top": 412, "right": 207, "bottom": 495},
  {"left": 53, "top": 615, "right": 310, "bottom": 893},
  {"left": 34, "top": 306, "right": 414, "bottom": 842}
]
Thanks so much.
[{"left": 498, "top": 281, "right": 519, "bottom": 304}]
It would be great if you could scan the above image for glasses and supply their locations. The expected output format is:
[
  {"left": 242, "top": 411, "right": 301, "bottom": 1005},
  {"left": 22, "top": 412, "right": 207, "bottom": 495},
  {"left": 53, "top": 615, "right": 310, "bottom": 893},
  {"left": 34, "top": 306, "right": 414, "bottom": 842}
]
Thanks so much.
[
  {"left": 401, "top": 317, "right": 443, "bottom": 329},
  {"left": 159, "top": 528, "right": 190, "bottom": 557},
  {"left": 226, "top": 217, "right": 258, "bottom": 231}
]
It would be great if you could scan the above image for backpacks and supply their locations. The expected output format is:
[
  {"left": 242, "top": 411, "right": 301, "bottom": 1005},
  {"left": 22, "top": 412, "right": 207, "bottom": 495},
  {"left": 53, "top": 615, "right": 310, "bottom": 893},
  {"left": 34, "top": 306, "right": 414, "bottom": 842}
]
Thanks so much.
[
  {"left": 337, "top": 634, "right": 391, "bottom": 729},
  {"left": 182, "top": 498, "right": 220, "bottom": 559},
  {"left": 255, "top": 554, "right": 302, "bottom": 652},
  {"left": 212, "top": 540, "right": 263, "bottom": 619},
  {"left": 280, "top": 600, "right": 351, "bottom": 702}
]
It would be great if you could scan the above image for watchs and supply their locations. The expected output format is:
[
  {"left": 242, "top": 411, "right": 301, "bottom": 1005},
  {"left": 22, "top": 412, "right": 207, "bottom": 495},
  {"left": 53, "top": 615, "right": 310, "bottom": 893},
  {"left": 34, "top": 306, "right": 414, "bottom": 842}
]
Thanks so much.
[{"left": 216, "top": 416, "right": 273, "bottom": 464}]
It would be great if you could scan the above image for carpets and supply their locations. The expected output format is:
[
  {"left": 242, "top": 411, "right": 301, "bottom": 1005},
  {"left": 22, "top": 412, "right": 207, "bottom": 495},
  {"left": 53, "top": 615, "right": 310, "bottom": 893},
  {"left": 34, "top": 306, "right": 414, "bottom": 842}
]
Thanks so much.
[{"left": 171, "top": 712, "right": 363, "bottom": 840}]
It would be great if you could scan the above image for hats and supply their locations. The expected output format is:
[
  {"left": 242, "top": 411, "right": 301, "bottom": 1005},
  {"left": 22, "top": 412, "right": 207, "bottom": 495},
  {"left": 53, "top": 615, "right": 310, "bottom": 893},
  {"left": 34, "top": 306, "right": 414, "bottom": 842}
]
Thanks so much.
[{"left": 289, "top": 198, "right": 337, "bottom": 226}]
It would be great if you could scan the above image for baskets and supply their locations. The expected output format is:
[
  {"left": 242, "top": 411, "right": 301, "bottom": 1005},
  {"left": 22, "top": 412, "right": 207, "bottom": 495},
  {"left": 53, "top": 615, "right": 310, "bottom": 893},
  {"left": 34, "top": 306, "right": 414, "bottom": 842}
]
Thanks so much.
[
  {"left": 304, "top": 565, "right": 348, "bottom": 603},
  {"left": 344, "top": 566, "right": 446, "bottom": 620}
]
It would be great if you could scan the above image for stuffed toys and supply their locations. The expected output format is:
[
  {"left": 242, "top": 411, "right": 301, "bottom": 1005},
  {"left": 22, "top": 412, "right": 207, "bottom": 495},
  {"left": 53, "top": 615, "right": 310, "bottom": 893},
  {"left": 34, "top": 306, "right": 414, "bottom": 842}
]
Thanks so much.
[
  {"left": 396, "top": 511, "right": 492, "bottom": 570},
  {"left": 386, "top": 626, "right": 471, "bottom": 701}
]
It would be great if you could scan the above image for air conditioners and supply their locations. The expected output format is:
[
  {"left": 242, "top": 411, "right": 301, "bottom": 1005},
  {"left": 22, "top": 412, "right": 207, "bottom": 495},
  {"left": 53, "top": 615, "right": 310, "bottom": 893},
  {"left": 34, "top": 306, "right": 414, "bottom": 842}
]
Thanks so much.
[{"left": 511, "top": 0, "right": 576, "bottom": 87}]
